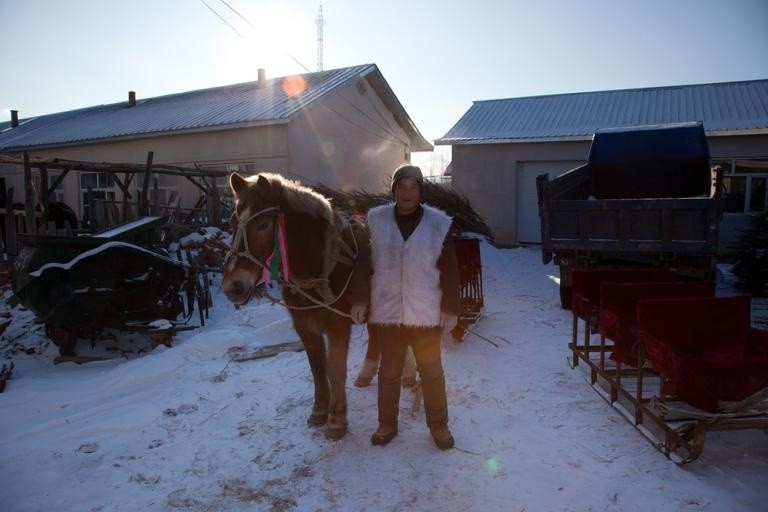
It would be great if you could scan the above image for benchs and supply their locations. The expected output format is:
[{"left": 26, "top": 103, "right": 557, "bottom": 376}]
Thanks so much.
[{"left": 562, "top": 263, "right": 767, "bottom": 465}]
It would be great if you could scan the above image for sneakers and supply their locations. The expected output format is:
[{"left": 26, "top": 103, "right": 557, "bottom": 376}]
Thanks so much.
[
  {"left": 430, "top": 423, "right": 454, "bottom": 449},
  {"left": 372, "top": 425, "right": 397, "bottom": 446}
]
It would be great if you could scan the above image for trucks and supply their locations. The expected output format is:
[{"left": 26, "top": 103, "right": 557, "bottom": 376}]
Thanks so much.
[{"left": 536, "top": 121, "right": 722, "bottom": 310}]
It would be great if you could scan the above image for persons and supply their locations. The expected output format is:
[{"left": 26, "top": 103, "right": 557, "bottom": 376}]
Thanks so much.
[{"left": 351, "top": 165, "right": 460, "bottom": 449}]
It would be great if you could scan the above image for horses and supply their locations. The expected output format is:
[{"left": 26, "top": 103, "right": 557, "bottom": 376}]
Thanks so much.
[{"left": 216, "top": 170, "right": 416, "bottom": 443}]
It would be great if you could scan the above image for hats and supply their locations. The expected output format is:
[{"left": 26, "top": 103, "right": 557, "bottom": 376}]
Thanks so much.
[{"left": 391, "top": 165, "right": 425, "bottom": 204}]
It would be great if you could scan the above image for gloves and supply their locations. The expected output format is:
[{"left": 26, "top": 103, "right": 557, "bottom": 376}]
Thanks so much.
[
  {"left": 440, "top": 313, "right": 458, "bottom": 335},
  {"left": 351, "top": 305, "right": 366, "bottom": 325}
]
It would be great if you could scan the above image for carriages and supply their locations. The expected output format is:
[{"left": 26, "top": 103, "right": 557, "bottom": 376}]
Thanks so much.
[{"left": 219, "top": 171, "right": 490, "bottom": 440}]
